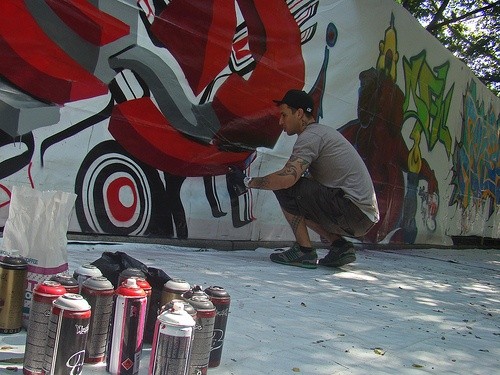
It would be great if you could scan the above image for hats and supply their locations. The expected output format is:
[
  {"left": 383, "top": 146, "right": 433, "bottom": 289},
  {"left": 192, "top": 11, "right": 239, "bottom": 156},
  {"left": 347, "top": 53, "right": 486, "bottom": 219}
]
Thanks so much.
[{"left": 272, "top": 90, "right": 314, "bottom": 116}]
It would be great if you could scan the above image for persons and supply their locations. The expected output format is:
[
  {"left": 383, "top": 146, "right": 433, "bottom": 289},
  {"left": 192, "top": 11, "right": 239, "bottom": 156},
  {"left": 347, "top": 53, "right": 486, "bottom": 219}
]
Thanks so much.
[{"left": 226, "top": 90, "right": 381, "bottom": 268}]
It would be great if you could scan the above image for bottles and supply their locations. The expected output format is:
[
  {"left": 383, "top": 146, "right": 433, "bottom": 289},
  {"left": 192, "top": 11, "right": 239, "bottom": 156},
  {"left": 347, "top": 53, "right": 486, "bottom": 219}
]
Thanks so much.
[
  {"left": 0, "top": 249, "right": 29, "bottom": 334},
  {"left": 228, "top": 167, "right": 248, "bottom": 196},
  {"left": 23, "top": 264, "right": 231, "bottom": 375}
]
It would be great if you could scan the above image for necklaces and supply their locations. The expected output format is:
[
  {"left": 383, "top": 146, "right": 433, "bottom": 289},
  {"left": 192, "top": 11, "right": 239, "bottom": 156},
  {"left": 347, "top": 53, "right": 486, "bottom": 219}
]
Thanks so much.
[{"left": 303, "top": 122, "right": 316, "bottom": 131}]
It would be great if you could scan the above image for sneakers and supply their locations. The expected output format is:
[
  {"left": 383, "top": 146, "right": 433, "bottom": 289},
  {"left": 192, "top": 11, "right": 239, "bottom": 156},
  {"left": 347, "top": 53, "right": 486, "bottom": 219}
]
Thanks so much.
[
  {"left": 318, "top": 240, "right": 356, "bottom": 266},
  {"left": 270, "top": 243, "right": 318, "bottom": 269}
]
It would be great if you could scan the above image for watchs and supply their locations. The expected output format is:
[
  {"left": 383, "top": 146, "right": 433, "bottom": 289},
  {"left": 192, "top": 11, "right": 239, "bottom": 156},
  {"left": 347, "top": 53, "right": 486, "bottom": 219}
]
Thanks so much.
[{"left": 244, "top": 177, "right": 252, "bottom": 189}]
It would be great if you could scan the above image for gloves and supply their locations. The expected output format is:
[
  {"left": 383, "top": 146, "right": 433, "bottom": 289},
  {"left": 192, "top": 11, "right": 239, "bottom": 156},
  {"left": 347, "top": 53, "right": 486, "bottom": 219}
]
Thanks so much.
[{"left": 227, "top": 164, "right": 246, "bottom": 188}]
